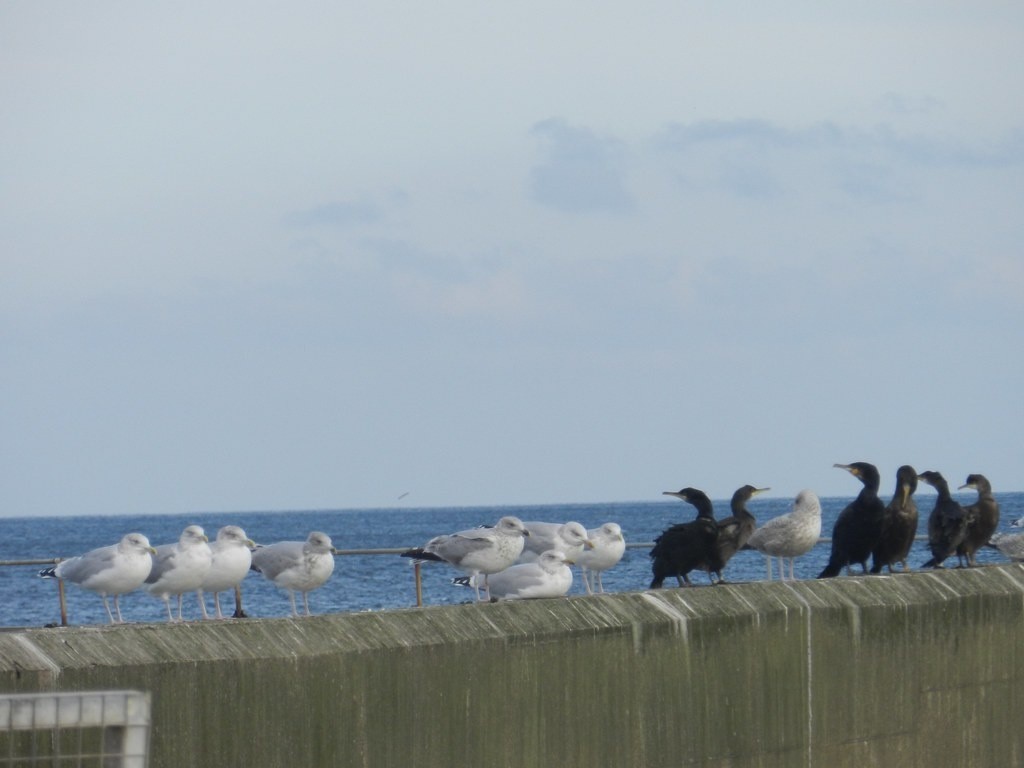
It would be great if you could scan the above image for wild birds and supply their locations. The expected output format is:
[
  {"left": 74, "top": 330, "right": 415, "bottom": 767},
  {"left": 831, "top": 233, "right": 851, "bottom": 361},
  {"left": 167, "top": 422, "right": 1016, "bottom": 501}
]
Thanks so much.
[
  {"left": 649, "top": 462, "right": 1000, "bottom": 590},
  {"left": 37, "top": 524, "right": 338, "bottom": 627},
  {"left": 400, "top": 516, "right": 626, "bottom": 603}
]
